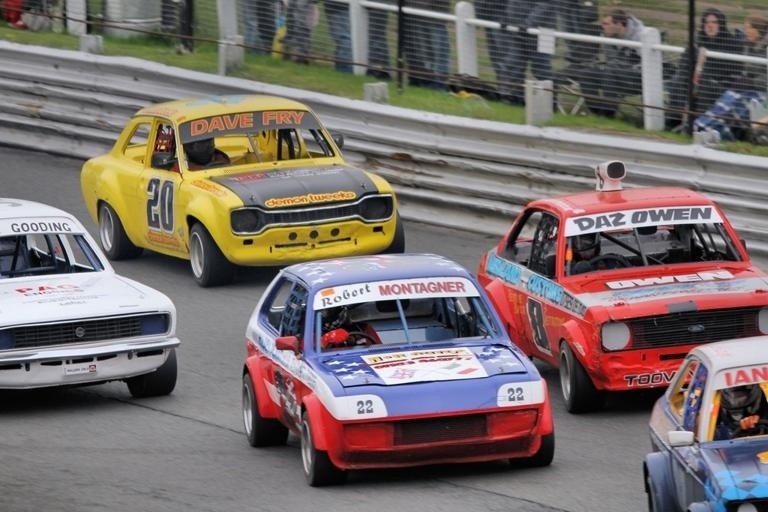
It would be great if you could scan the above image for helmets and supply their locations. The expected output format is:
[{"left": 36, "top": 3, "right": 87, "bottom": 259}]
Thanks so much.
[
  {"left": 182, "top": 137, "right": 215, "bottom": 166},
  {"left": 571, "top": 233, "right": 601, "bottom": 262},
  {"left": 315, "top": 306, "right": 351, "bottom": 334},
  {"left": 719, "top": 383, "right": 761, "bottom": 430}
]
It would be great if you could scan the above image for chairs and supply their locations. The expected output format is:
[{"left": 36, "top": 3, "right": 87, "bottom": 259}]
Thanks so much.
[{"left": 0, "top": 241, "right": 30, "bottom": 278}]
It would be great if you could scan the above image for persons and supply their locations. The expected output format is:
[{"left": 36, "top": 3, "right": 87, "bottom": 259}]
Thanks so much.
[
  {"left": 170, "top": 138, "right": 230, "bottom": 173},
  {"left": 713, "top": 383, "right": 768, "bottom": 440},
  {"left": 570, "top": 232, "right": 625, "bottom": 274},
  {"left": 322, "top": 306, "right": 366, "bottom": 349}
]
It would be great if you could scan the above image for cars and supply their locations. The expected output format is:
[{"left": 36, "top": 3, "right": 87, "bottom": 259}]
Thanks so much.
[
  {"left": 81, "top": 95, "right": 403, "bottom": 287},
  {"left": 1, "top": 199, "right": 178, "bottom": 398},
  {"left": 477, "top": 163, "right": 763, "bottom": 413},
  {"left": 642, "top": 334, "right": 763, "bottom": 511},
  {"left": 240, "top": 253, "right": 554, "bottom": 486}
]
